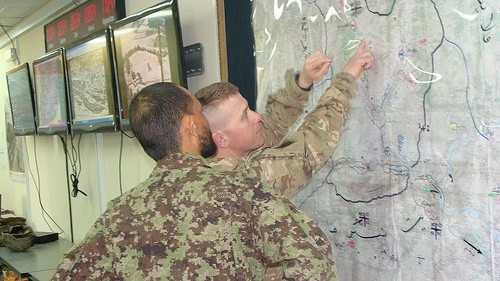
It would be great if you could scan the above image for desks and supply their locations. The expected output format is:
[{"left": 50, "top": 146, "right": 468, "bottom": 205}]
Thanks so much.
[
  {"left": 29, "top": 269, "right": 58, "bottom": 281},
  {"left": 0, "top": 231, "right": 78, "bottom": 278}
]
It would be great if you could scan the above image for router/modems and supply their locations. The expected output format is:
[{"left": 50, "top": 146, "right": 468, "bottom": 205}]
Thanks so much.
[{"left": 35, "top": 231, "right": 59, "bottom": 246}]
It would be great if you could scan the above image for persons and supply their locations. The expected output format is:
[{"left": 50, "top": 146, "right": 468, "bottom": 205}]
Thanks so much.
[
  {"left": 50, "top": 80, "right": 336, "bottom": 281},
  {"left": 193, "top": 39, "right": 374, "bottom": 201}
]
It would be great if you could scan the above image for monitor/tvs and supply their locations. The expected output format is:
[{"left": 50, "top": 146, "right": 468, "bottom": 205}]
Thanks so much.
[
  {"left": 31, "top": 45, "right": 71, "bottom": 135},
  {"left": 5, "top": 61, "right": 36, "bottom": 136},
  {"left": 109, "top": 0, "right": 189, "bottom": 133},
  {"left": 63, "top": 25, "right": 121, "bottom": 135}
]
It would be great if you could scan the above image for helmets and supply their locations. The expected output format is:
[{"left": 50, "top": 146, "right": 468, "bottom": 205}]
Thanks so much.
[{"left": 1, "top": 224, "right": 35, "bottom": 252}]
